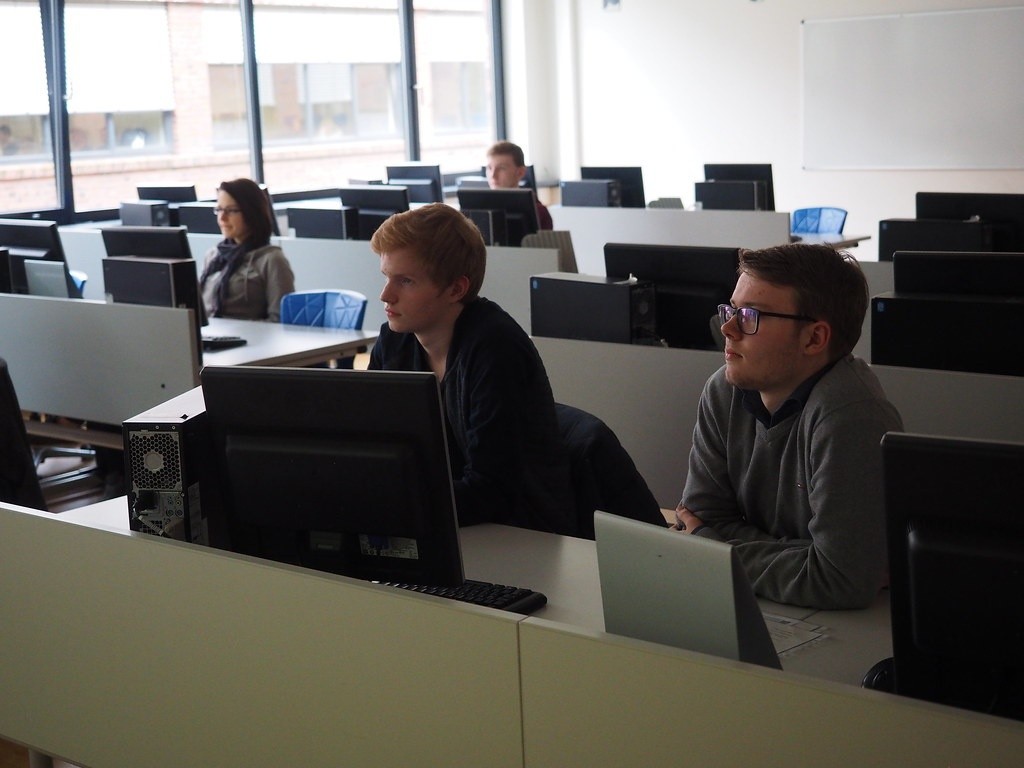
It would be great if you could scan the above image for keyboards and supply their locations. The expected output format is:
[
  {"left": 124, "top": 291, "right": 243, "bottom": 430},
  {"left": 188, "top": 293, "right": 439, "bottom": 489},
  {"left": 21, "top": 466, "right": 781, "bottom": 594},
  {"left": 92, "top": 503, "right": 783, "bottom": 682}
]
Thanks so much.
[
  {"left": 199, "top": 333, "right": 247, "bottom": 350},
  {"left": 369, "top": 580, "right": 548, "bottom": 614}
]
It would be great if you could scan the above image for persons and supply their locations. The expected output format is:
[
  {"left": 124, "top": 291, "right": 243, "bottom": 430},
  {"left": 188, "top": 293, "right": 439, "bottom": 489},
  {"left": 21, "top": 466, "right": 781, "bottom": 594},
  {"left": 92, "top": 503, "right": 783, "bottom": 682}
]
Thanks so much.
[
  {"left": 485, "top": 140, "right": 553, "bottom": 229},
  {"left": 364, "top": 204, "right": 557, "bottom": 525},
  {"left": 667, "top": 245, "right": 901, "bottom": 612},
  {"left": 200, "top": 177, "right": 295, "bottom": 324}
]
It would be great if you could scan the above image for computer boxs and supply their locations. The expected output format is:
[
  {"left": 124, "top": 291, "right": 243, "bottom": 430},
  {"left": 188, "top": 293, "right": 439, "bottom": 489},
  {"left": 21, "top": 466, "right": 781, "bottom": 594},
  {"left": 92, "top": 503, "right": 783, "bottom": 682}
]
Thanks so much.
[
  {"left": 0, "top": 356, "right": 47, "bottom": 513},
  {"left": 869, "top": 290, "right": 1024, "bottom": 378},
  {"left": 878, "top": 217, "right": 994, "bottom": 262},
  {"left": 0, "top": 248, "right": 12, "bottom": 294},
  {"left": 100, "top": 180, "right": 767, "bottom": 553}
]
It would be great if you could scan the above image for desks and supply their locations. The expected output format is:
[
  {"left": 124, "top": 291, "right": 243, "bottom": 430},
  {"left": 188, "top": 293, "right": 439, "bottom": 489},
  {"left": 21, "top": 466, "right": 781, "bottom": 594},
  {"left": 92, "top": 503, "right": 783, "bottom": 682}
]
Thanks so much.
[{"left": 1, "top": 188, "right": 1024, "bottom": 768}]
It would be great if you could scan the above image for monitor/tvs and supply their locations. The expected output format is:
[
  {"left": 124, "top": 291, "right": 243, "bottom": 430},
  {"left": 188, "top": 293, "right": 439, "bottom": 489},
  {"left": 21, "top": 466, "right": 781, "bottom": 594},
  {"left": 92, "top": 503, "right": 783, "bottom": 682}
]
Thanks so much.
[
  {"left": 603, "top": 243, "right": 742, "bottom": 353},
  {"left": 338, "top": 162, "right": 776, "bottom": 246},
  {"left": 100, "top": 225, "right": 210, "bottom": 325},
  {"left": 138, "top": 186, "right": 197, "bottom": 227},
  {"left": 0, "top": 218, "right": 69, "bottom": 296},
  {"left": 258, "top": 185, "right": 282, "bottom": 236},
  {"left": 893, "top": 192, "right": 1024, "bottom": 305},
  {"left": 879, "top": 433, "right": 1024, "bottom": 723},
  {"left": 201, "top": 366, "right": 465, "bottom": 586}
]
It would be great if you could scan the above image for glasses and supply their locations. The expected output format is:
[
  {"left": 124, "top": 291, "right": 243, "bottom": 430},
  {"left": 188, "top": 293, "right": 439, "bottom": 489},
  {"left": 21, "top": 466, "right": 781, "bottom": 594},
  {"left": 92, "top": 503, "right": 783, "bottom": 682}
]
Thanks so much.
[
  {"left": 213, "top": 206, "right": 244, "bottom": 216},
  {"left": 718, "top": 304, "right": 818, "bottom": 335}
]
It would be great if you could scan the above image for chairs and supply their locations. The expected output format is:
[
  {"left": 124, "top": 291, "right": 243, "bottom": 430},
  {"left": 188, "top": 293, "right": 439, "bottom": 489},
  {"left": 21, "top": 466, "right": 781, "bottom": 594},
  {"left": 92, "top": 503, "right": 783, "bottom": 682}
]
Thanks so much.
[
  {"left": 791, "top": 207, "right": 848, "bottom": 235},
  {"left": 277, "top": 288, "right": 367, "bottom": 369},
  {"left": 552, "top": 400, "right": 609, "bottom": 541}
]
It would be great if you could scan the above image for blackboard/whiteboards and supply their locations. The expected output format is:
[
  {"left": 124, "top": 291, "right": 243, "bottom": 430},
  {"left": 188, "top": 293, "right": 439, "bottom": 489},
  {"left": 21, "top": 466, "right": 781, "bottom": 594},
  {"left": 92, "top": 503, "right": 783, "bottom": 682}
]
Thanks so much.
[{"left": 801, "top": 6, "right": 1024, "bottom": 171}]
